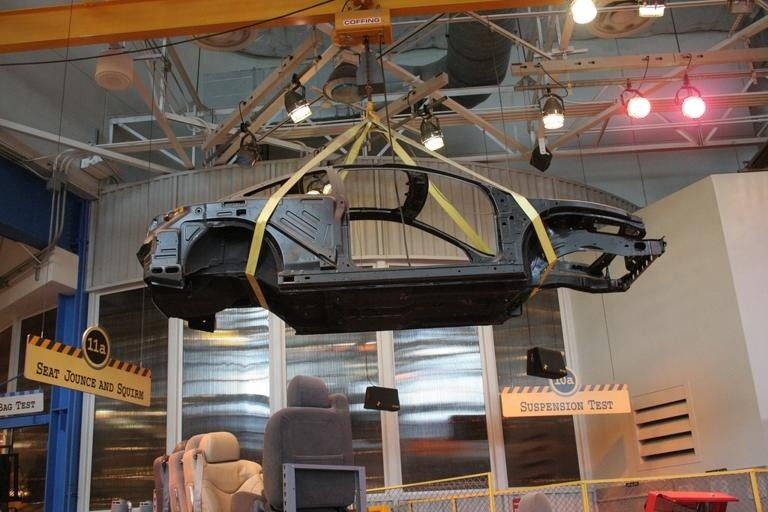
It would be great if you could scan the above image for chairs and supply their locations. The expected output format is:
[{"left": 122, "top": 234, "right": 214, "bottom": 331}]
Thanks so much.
[{"left": 153, "top": 376, "right": 367, "bottom": 512}]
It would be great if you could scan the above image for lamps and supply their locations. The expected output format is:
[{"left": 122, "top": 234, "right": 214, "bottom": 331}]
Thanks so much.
[
  {"left": 672, "top": 82, "right": 704, "bottom": 123},
  {"left": 568, "top": 2, "right": 600, "bottom": 27},
  {"left": 282, "top": 89, "right": 313, "bottom": 124},
  {"left": 537, "top": 88, "right": 568, "bottom": 133},
  {"left": 617, "top": 82, "right": 654, "bottom": 120},
  {"left": 417, "top": 117, "right": 447, "bottom": 152}
]
